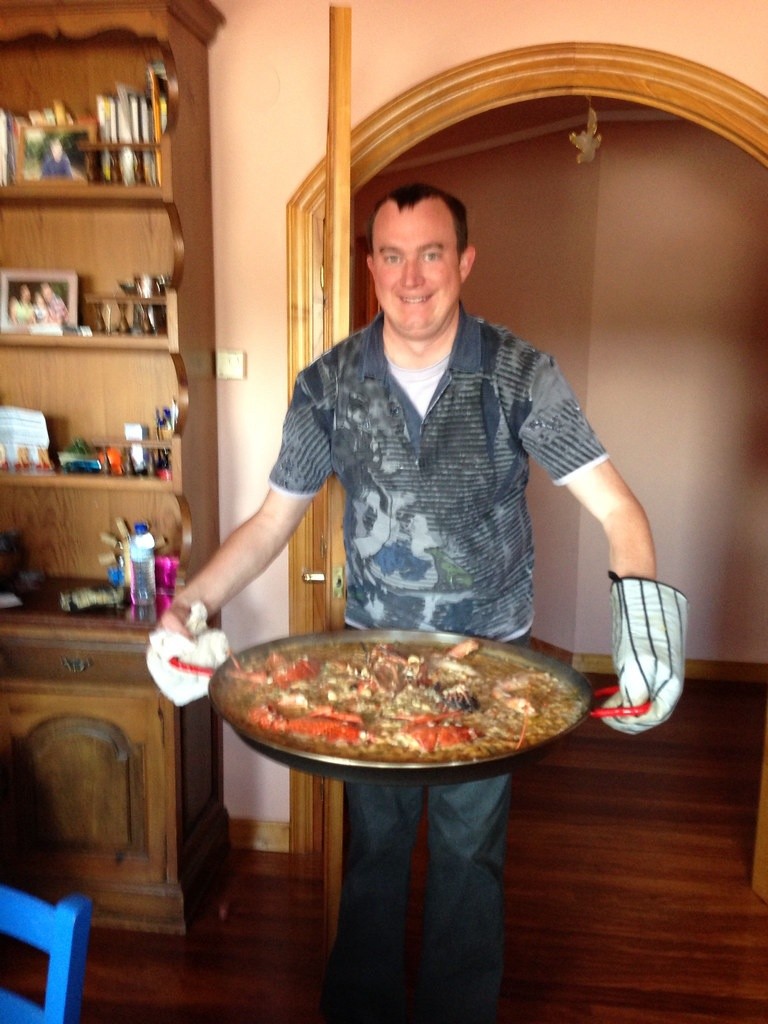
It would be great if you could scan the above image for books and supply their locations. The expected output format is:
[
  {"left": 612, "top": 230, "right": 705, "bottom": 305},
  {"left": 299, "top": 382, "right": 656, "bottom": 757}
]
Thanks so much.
[{"left": 0, "top": 62, "right": 167, "bottom": 186}]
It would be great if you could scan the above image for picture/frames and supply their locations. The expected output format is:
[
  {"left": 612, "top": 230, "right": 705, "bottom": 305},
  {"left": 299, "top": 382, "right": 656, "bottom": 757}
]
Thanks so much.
[
  {"left": 0, "top": 268, "right": 78, "bottom": 335},
  {"left": 16, "top": 123, "right": 98, "bottom": 186}
]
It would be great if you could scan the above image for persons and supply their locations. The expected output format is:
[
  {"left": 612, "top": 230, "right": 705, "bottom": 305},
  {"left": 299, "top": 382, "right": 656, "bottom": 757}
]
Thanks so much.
[
  {"left": 8, "top": 282, "right": 68, "bottom": 326},
  {"left": 41, "top": 136, "right": 74, "bottom": 179},
  {"left": 145, "top": 184, "right": 689, "bottom": 1023}
]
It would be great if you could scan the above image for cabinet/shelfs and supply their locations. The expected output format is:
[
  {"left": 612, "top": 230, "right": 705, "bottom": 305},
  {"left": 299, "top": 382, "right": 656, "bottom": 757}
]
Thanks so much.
[{"left": 0, "top": 0, "right": 229, "bottom": 935}]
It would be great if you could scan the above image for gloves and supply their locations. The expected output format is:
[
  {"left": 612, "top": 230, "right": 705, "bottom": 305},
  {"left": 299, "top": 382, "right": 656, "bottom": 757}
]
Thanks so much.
[{"left": 597, "top": 570, "right": 688, "bottom": 734}]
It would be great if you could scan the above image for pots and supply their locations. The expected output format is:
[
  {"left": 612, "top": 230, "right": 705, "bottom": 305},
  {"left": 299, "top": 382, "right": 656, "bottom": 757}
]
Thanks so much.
[{"left": 167, "top": 628, "right": 651, "bottom": 784}]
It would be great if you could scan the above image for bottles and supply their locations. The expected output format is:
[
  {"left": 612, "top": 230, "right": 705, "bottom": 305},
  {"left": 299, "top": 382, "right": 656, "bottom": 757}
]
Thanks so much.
[{"left": 128, "top": 523, "right": 156, "bottom": 605}]
